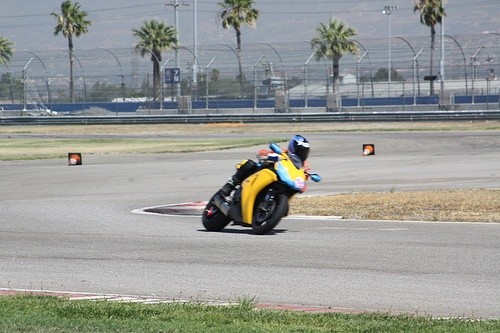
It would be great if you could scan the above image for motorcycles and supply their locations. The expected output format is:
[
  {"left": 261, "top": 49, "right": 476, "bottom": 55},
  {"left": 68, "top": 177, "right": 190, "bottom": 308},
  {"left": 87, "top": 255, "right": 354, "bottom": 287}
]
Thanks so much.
[{"left": 201, "top": 143, "right": 320, "bottom": 234}]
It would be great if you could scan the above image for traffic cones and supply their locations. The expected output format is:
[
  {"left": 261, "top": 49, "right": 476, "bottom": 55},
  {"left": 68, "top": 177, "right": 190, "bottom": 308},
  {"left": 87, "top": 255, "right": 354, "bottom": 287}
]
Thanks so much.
[
  {"left": 363, "top": 143, "right": 375, "bottom": 157},
  {"left": 68, "top": 152, "right": 82, "bottom": 165}
]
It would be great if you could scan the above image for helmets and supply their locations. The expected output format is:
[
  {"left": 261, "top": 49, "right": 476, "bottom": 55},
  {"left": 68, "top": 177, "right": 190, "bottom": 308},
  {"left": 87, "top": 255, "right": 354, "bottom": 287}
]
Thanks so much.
[{"left": 289, "top": 133, "right": 310, "bottom": 160}]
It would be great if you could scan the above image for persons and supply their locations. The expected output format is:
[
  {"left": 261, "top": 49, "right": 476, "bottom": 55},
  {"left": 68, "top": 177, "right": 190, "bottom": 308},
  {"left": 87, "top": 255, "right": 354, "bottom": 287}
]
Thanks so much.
[{"left": 219, "top": 133, "right": 311, "bottom": 218}]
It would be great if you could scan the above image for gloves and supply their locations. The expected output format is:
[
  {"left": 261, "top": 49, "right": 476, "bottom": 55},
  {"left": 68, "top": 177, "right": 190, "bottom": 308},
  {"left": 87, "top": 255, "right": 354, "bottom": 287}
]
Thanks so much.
[{"left": 267, "top": 152, "right": 281, "bottom": 164}]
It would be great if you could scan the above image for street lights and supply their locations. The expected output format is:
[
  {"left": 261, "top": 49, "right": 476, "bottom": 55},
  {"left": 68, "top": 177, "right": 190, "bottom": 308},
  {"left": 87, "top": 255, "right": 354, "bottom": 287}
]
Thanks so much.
[{"left": 6, "top": 44, "right": 485, "bottom": 110}]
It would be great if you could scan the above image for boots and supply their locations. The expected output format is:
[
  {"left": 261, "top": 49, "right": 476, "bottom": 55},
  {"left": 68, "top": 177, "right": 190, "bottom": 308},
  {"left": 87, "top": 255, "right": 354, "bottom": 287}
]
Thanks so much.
[{"left": 220, "top": 175, "right": 240, "bottom": 196}]
